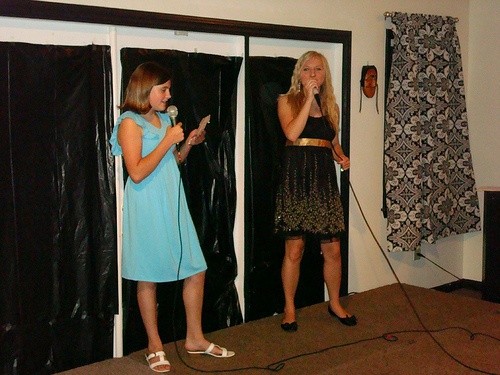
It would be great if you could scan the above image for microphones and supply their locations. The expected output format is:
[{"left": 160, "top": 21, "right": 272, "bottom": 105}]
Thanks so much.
[
  {"left": 312, "top": 87, "right": 322, "bottom": 109},
  {"left": 166, "top": 105, "right": 182, "bottom": 163}
]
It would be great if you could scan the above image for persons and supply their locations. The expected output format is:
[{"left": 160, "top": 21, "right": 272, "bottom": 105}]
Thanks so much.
[
  {"left": 275, "top": 51, "right": 358, "bottom": 331},
  {"left": 109, "top": 61, "right": 236, "bottom": 372}
]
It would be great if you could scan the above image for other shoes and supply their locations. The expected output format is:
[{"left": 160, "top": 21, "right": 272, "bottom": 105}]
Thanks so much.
[
  {"left": 328, "top": 304, "right": 357, "bottom": 326},
  {"left": 281, "top": 321, "right": 297, "bottom": 332}
]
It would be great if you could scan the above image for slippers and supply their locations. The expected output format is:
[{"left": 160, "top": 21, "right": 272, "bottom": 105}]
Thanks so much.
[
  {"left": 144, "top": 351, "right": 171, "bottom": 372},
  {"left": 186, "top": 342, "right": 235, "bottom": 358}
]
